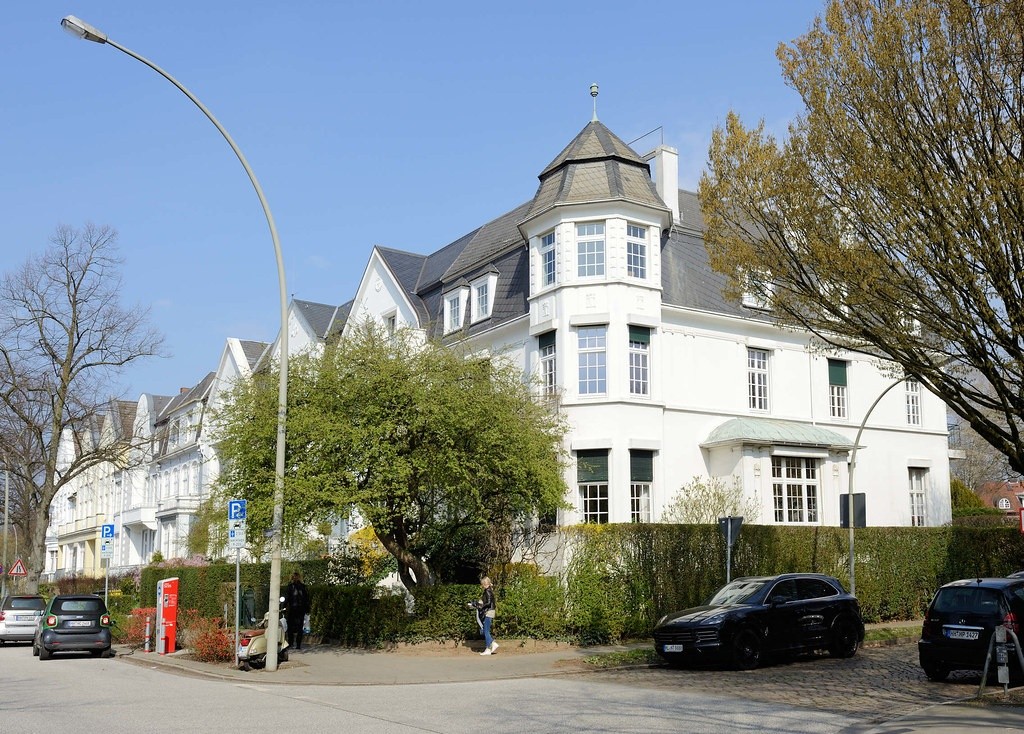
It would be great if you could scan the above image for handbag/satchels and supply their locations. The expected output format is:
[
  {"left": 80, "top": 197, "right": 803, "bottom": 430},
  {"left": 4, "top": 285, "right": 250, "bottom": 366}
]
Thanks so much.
[
  {"left": 303, "top": 615, "right": 311, "bottom": 633},
  {"left": 280, "top": 615, "right": 288, "bottom": 633}
]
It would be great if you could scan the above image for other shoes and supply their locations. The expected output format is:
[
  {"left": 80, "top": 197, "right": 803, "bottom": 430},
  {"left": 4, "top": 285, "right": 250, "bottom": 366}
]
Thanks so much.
[{"left": 481, "top": 642, "right": 499, "bottom": 655}]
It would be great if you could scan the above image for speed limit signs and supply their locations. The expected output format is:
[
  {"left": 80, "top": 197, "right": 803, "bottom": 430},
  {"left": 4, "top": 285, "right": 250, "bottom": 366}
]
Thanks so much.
[{"left": 8, "top": 559, "right": 29, "bottom": 577}]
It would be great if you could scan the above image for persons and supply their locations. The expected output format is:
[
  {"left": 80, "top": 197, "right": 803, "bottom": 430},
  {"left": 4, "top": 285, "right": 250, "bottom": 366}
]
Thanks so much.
[
  {"left": 471, "top": 576, "right": 499, "bottom": 658},
  {"left": 277, "top": 570, "right": 313, "bottom": 650}
]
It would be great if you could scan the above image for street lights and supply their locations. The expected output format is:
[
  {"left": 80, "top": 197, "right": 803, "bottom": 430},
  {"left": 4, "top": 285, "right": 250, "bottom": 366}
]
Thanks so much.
[{"left": 60, "top": 16, "right": 291, "bottom": 674}]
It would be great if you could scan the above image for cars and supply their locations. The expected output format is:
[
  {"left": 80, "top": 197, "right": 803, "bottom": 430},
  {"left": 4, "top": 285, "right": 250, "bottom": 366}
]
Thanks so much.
[
  {"left": 31, "top": 592, "right": 114, "bottom": 660},
  {"left": 649, "top": 569, "right": 868, "bottom": 671},
  {"left": 914, "top": 576, "right": 1023, "bottom": 688},
  {"left": 0, "top": 594, "right": 47, "bottom": 646}
]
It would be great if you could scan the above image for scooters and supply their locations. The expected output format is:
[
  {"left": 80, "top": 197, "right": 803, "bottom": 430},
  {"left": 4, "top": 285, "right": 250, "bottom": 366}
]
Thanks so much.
[{"left": 237, "top": 596, "right": 291, "bottom": 672}]
